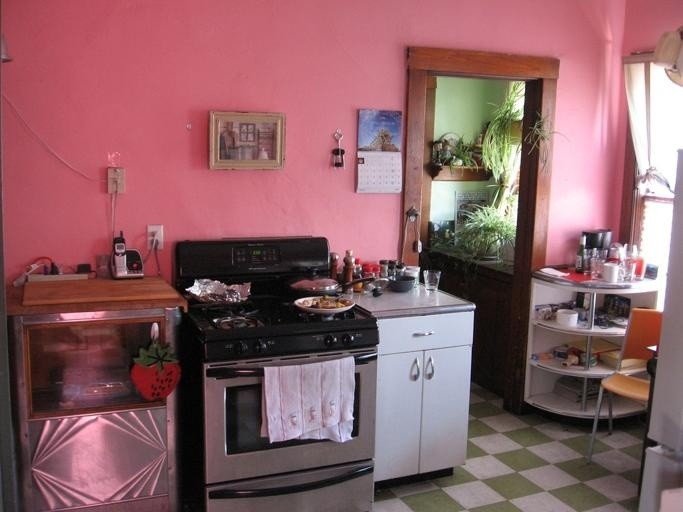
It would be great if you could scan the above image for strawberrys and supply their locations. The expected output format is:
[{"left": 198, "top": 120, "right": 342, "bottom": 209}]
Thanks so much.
[{"left": 128, "top": 344, "right": 181, "bottom": 403}]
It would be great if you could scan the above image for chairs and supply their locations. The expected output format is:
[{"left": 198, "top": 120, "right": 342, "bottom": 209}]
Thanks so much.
[{"left": 583, "top": 304, "right": 665, "bottom": 468}]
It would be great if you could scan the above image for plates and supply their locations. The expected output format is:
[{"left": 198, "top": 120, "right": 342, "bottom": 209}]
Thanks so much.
[{"left": 294, "top": 296, "right": 356, "bottom": 315}]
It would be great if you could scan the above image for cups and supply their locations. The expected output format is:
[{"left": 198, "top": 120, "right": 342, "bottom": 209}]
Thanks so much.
[
  {"left": 423, "top": 269, "right": 441, "bottom": 292},
  {"left": 582, "top": 243, "right": 647, "bottom": 283}
]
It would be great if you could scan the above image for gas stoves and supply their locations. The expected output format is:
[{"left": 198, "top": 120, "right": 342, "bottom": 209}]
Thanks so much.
[{"left": 189, "top": 293, "right": 379, "bottom": 362}]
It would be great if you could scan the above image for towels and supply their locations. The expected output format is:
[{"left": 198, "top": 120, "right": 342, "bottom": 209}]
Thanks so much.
[{"left": 259, "top": 356, "right": 355, "bottom": 447}]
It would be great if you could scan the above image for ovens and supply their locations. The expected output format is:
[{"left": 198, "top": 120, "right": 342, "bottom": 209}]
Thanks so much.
[{"left": 200, "top": 345, "right": 380, "bottom": 511}]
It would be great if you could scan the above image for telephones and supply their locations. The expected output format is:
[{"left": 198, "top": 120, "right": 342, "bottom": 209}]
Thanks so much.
[{"left": 110, "top": 231, "right": 144, "bottom": 279}]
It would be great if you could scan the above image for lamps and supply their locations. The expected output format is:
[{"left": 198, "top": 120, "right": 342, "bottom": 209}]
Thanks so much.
[{"left": 649, "top": 23, "right": 682, "bottom": 90}]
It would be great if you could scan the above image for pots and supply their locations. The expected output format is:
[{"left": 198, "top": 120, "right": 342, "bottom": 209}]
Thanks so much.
[{"left": 289, "top": 267, "right": 376, "bottom": 297}]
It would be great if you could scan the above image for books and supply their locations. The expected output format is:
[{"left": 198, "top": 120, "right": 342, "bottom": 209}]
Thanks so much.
[
  {"left": 572, "top": 338, "right": 649, "bottom": 370},
  {"left": 553, "top": 376, "right": 618, "bottom": 402}
]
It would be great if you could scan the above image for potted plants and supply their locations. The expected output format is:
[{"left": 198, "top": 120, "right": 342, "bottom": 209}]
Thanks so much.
[
  {"left": 440, "top": 134, "right": 479, "bottom": 178},
  {"left": 476, "top": 81, "right": 526, "bottom": 190},
  {"left": 437, "top": 200, "right": 517, "bottom": 271}
]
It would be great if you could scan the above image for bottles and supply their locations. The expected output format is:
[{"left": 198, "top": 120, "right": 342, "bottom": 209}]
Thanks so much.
[
  {"left": 575, "top": 235, "right": 587, "bottom": 273},
  {"left": 330, "top": 249, "right": 420, "bottom": 297},
  {"left": 257, "top": 148, "right": 268, "bottom": 160}
]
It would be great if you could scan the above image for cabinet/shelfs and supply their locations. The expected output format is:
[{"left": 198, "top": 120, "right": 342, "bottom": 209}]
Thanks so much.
[
  {"left": 423, "top": 249, "right": 512, "bottom": 402},
  {"left": 518, "top": 260, "right": 660, "bottom": 422},
  {"left": 335, "top": 276, "right": 476, "bottom": 491}
]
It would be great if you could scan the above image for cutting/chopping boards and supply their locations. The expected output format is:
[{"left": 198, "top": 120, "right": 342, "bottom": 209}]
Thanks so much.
[{"left": 22, "top": 278, "right": 180, "bottom": 306}]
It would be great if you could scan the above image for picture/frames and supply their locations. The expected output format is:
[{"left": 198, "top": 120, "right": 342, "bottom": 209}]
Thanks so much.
[{"left": 209, "top": 107, "right": 286, "bottom": 172}]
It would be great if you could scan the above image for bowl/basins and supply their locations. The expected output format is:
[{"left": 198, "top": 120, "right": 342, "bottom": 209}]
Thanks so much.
[{"left": 556, "top": 308, "right": 578, "bottom": 327}]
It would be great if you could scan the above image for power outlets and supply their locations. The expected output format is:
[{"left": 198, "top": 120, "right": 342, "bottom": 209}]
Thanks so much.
[
  {"left": 106, "top": 166, "right": 127, "bottom": 195},
  {"left": 144, "top": 222, "right": 165, "bottom": 253}
]
